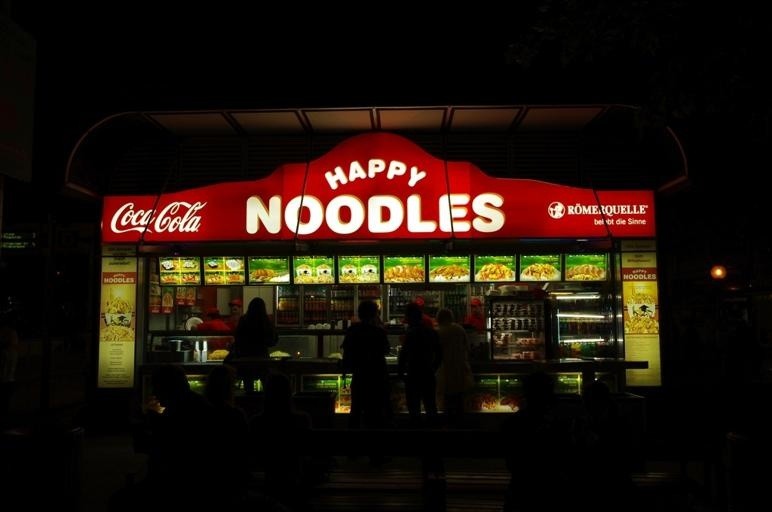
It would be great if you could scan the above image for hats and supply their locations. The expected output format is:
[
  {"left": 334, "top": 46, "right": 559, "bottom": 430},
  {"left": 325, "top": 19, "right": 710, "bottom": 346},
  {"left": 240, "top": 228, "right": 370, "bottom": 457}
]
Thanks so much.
[
  {"left": 207, "top": 307, "right": 219, "bottom": 316},
  {"left": 229, "top": 297, "right": 242, "bottom": 305},
  {"left": 413, "top": 296, "right": 426, "bottom": 307},
  {"left": 471, "top": 298, "right": 481, "bottom": 306}
]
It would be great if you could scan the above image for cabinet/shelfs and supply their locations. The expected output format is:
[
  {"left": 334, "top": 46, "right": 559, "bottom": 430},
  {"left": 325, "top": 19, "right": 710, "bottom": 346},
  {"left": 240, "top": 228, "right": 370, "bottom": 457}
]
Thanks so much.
[{"left": 272, "top": 286, "right": 616, "bottom": 361}]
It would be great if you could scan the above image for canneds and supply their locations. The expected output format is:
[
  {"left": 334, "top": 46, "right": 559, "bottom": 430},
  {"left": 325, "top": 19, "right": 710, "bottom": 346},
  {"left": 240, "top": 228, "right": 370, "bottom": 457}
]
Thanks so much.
[{"left": 492, "top": 303, "right": 543, "bottom": 332}]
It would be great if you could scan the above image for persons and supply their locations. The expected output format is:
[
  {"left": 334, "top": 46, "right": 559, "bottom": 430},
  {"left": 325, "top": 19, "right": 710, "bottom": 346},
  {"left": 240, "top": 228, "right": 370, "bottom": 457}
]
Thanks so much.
[
  {"left": 189, "top": 365, "right": 247, "bottom": 434},
  {"left": 143, "top": 396, "right": 160, "bottom": 413},
  {"left": 246, "top": 374, "right": 314, "bottom": 433},
  {"left": 155, "top": 370, "right": 202, "bottom": 414},
  {"left": 397, "top": 299, "right": 469, "bottom": 417},
  {"left": 342, "top": 300, "right": 391, "bottom": 414},
  {"left": 464, "top": 299, "right": 486, "bottom": 334},
  {"left": 224, "top": 296, "right": 277, "bottom": 392},
  {"left": 196, "top": 299, "right": 243, "bottom": 350}
]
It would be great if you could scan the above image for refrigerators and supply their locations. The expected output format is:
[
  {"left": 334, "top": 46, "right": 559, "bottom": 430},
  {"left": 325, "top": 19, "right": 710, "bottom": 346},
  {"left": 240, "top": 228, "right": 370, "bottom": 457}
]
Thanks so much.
[{"left": 544, "top": 294, "right": 612, "bottom": 361}]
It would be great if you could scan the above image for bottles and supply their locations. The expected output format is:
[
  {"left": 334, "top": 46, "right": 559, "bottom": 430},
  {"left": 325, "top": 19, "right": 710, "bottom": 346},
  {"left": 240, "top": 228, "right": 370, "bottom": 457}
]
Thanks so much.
[{"left": 558, "top": 314, "right": 607, "bottom": 356}]
